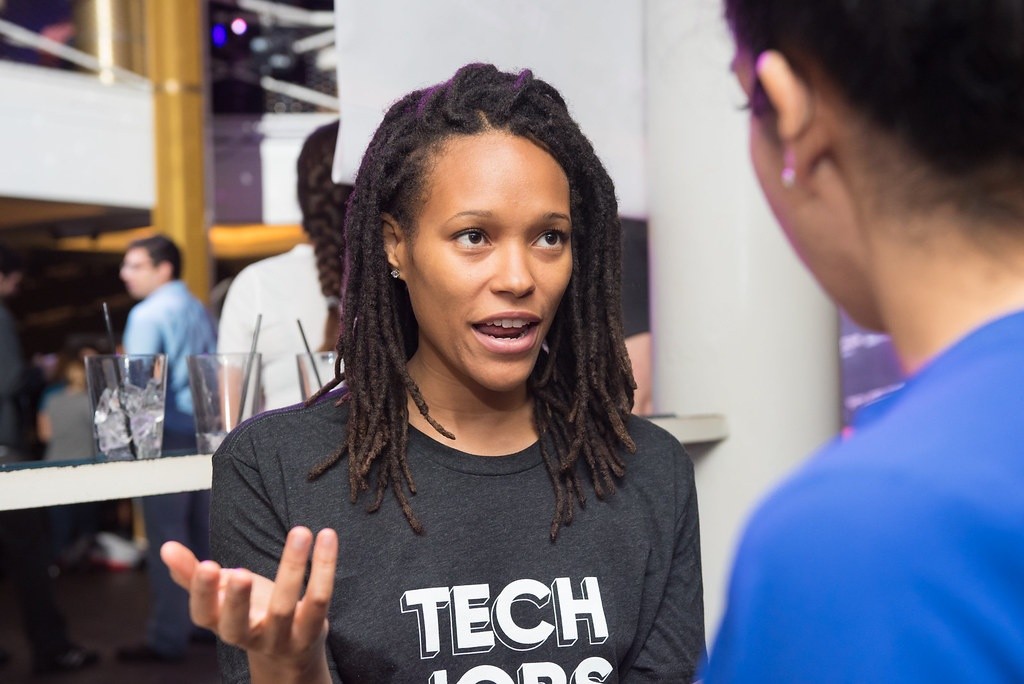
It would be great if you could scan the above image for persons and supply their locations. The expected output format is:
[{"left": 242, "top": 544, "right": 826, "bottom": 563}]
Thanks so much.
[
  {"left": 0, "top": 118, "right": 353, "bottom": 675},
  {"left": 699, "top": 1, "right": 1024, "bottom": 684},
  {"left": 161, "top": 65, "right": 708, "bottom": 684}
]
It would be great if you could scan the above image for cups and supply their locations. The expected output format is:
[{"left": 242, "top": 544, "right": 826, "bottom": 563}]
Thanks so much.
[
  {"left": 185, "top": 353, "right": 263, "bottom": 454},
  {"left": 85, "top": 354, "right": 168, "bottom": 459},
  {"left": 297, "top": 352, "right": 345, "bottom": 402}
]
